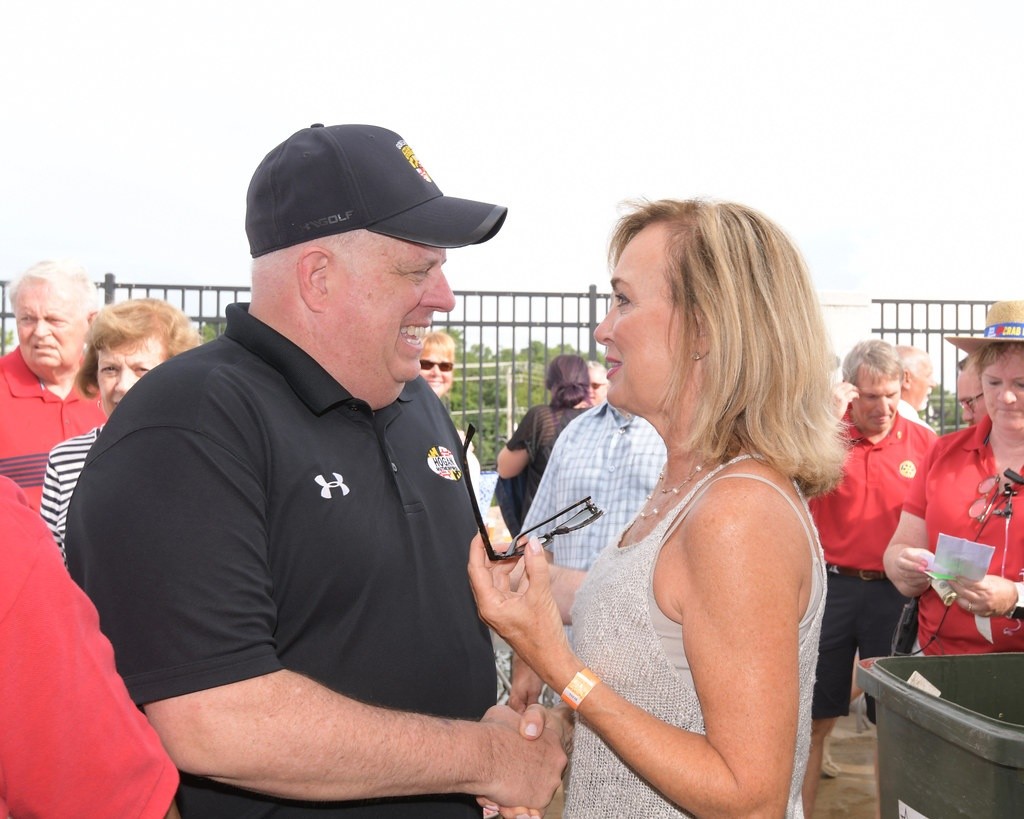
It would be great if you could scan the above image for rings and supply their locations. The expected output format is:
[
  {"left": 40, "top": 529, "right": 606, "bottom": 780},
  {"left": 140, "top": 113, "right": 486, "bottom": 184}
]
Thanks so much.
[{"left": 965, "top": 602, "right": 973, "bottom": 612}]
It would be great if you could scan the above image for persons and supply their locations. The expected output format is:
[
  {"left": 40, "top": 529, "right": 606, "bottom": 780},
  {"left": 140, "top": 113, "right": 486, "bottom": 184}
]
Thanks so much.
[
  {"left": 587, "top": 360, "right": 609, "bottom": 407},
  {"left": 0, "top": 476, "right": 180, "bottom": 818},
  {"left": 508, "top": 405, "right": 669, "bottom": 716},
  {"left": 957, "top": 352, "right": 988, "bottom": 430},
  {"left": 39, "top": 298, "right": 205, "bottom": 572},
  {"left": 0, "top": 254, "right": 108, "bottom": 522},
  {"left": 64, "top": 122, "right": 573, "bottom": 819},
  {"left": 882, "top": 299, "right": 1024, "bottom": 658},
  {"left": 465, "top": 197, "right": 850, "bottom": 819},
  {"left": 420, "top": 333, "right": 480, "bottom": 522},
  {"left": 801, "top": 341, "right": 941, "bottom": 819},
  {"left": 895, "top": 345, "right": 939, "bottom": 435},
  {"left": 497, "top": 353, "right": 589, "bottom": 536}
]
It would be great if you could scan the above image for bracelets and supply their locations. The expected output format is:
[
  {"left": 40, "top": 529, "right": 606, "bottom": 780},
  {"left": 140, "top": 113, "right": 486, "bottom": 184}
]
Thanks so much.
[{"left": 561, "top": 666, "right": 602, "bottom": 712}]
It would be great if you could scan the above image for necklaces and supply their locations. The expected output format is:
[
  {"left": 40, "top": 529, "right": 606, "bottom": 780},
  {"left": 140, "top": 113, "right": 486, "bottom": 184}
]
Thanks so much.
[{"left": 640, "top": 449, "right": 708, "bottom": 520}]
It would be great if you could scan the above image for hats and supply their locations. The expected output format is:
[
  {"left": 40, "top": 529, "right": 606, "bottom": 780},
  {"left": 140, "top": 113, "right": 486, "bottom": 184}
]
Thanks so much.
[
  {"left": 944, "top": 302, "right": 1024, "bottom": 354},
  {"left": 245, "top": 123, "right": 508, "bottom": 259}
]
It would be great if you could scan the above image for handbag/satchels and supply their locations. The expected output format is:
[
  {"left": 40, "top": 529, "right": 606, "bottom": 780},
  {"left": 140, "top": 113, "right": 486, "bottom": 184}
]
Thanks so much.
[{"left": 891, "top": 596, "right": 919, "bottom": 657}]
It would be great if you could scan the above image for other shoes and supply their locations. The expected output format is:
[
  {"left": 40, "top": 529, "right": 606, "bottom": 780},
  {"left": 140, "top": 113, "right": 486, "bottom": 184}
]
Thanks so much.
[{"left": 821, "top": 755, "right": 840, "bottom": 779}]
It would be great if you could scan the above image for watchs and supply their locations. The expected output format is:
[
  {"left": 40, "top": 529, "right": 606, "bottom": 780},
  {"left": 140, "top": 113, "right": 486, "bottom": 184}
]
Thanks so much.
[{"left": 1005, "top": 582, "right": 1024, "bottom": 621}]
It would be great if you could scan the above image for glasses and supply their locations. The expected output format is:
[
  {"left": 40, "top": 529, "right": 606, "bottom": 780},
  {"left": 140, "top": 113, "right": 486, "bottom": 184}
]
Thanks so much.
[
  {"left": 419, "top": 359, "right": 453, "bottom": 373},
  {"left": 960, "top": 392, "right": 984, "bottom": 414},
  {"left": 591, "top": 383, "right": 606, "bottom": 390},
  {"left": 461, "top": 422, "right": 603, "bottom": 563}
]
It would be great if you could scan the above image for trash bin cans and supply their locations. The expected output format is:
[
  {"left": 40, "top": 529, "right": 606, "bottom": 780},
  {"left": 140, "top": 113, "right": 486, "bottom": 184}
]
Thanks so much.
[{"left": 856, "top": 652, "right": 1023, "bottom": 819}]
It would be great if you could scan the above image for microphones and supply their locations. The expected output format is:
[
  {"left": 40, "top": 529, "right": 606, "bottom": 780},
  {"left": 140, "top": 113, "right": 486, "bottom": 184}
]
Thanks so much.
[{"left": 1004, "top": 486, "right": 1014, "bottom": 497}]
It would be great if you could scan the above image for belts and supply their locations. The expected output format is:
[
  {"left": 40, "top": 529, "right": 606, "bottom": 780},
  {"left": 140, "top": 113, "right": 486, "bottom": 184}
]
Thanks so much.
[{"left": 836, "top": 565, "right": 888, "bottom": 580}]
